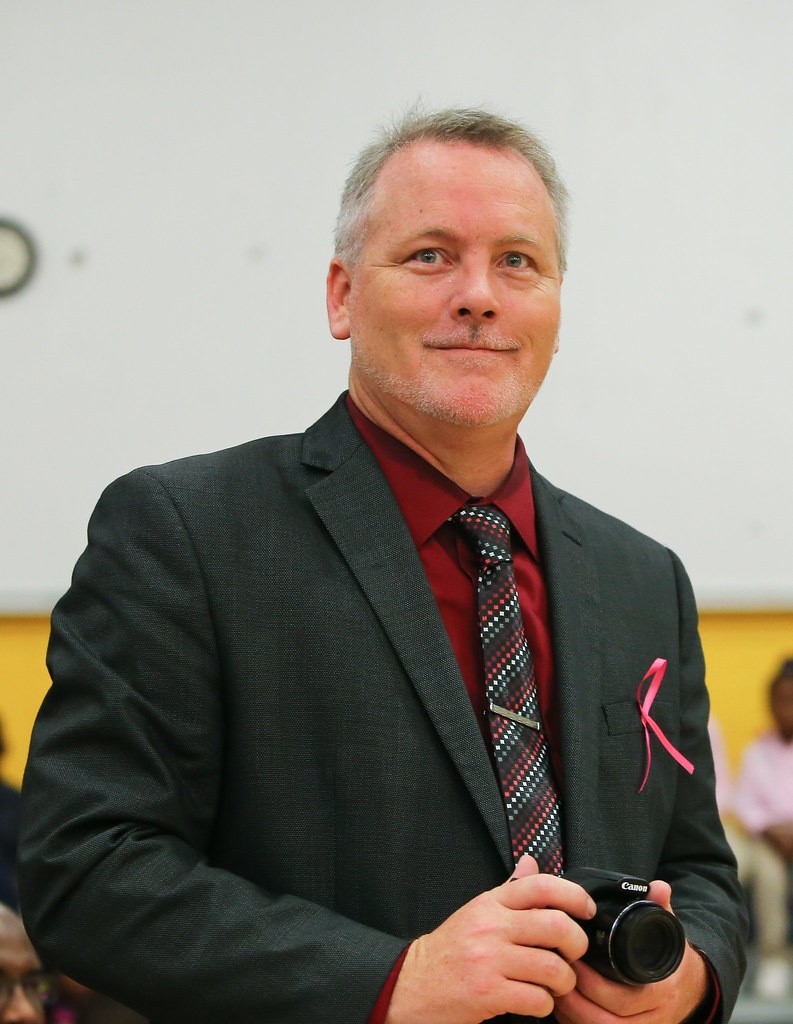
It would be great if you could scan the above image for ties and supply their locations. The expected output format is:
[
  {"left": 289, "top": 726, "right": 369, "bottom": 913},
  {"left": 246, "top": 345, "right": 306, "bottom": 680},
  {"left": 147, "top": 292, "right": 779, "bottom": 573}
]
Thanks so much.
[{"left": 442, "top": 503, "right": 564, "bottom": 875}]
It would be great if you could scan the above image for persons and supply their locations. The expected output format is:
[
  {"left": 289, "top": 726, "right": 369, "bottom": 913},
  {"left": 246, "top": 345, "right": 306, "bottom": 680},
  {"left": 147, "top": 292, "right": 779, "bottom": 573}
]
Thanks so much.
[
  {"left": 738, "top": 653, "right": 793, "bottom": 997},
  {"left": 14, "top": 105, "right": 751, "bottom": 1024}
]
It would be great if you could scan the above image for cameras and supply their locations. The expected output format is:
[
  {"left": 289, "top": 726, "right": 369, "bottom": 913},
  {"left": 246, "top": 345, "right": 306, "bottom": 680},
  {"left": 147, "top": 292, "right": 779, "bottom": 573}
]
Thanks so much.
[{"left": 548, "top": 867, "right": 685, "bottom": 988}]
